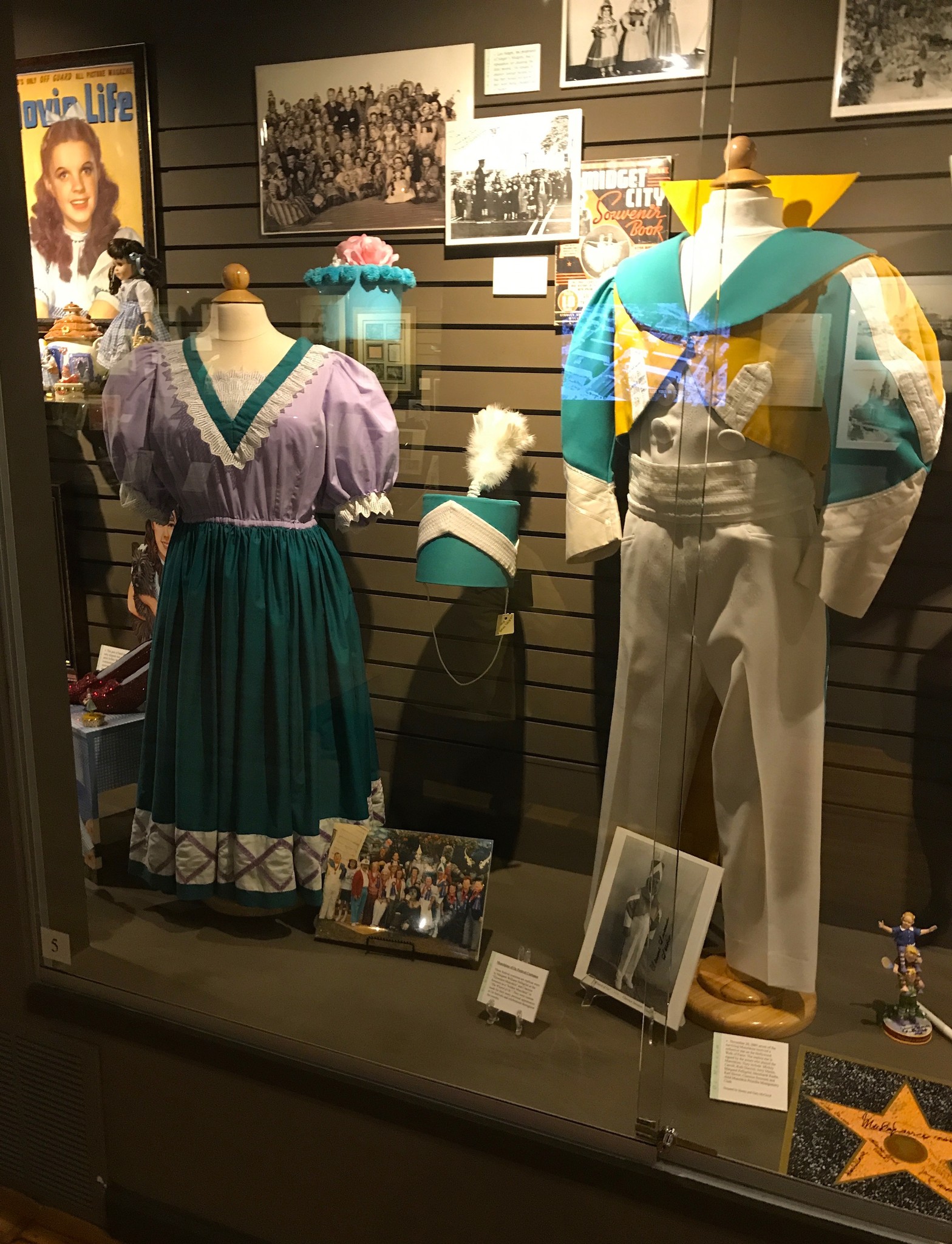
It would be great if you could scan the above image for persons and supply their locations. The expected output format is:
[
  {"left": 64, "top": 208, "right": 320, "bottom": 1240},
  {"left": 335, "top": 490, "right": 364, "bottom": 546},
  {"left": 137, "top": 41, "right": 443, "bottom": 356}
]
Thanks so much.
[
  {"left": 28, "top": 118, "right": 139, "bottom": 319},
  {"left": 95, "top": 238, "right": 172, "bottom": 370},
  {"left": 319, "top": 838, "right": 487, "bottom": 950},
  {"left": 100, "top": 264, "right": 399, "bottom": 916},
  {"left": 561, "top": 132, "right": 946, "bottom": 992},
  {"left": 260, "top": 0, "right": 952, "bottom": 226},
  {"left": 616, "top": 860, "right": 664, "bottom": 990},
  {"left": 878, "top": 912, "right": 938, "bottom": 1025}
]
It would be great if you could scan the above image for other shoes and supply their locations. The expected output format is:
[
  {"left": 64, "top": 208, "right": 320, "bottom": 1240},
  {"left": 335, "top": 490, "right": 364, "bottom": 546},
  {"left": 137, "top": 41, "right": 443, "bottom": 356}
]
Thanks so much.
[
  {"left": 615, "top": 978, "right": 622, "bottom": 990},
  {"left": 624, "top": 977, "right": 633, "bottom": 989}
]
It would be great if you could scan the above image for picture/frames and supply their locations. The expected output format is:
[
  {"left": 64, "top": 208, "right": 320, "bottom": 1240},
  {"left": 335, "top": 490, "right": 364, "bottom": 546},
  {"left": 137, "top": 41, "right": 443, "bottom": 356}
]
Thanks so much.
[
  {"left": 298, "top": 288, "right": 351, "bottom": 354},
  {"left": 835, "top": 270, "right": 952, "bottom": 452},
  {"left": 14, "top": 40, "right": 169, "bottom": 337},
  {"left": 352, "top": 304, "right": 417, "bottom": 398}
]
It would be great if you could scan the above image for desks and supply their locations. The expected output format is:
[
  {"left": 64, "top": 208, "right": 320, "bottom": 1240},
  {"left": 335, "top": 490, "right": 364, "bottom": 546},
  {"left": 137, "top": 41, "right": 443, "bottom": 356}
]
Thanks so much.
[{"left": 69, "top": 702, "right": 146, "bottom": 885}]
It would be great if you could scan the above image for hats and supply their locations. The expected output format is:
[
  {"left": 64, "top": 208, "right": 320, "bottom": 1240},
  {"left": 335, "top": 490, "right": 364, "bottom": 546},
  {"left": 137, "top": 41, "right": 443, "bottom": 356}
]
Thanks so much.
[
  {"left": 647, "top": 860, "right": 665, "bottom": 881},
  {"left": 416, "top": 402, "right": 536, "bottom": 588}
]
